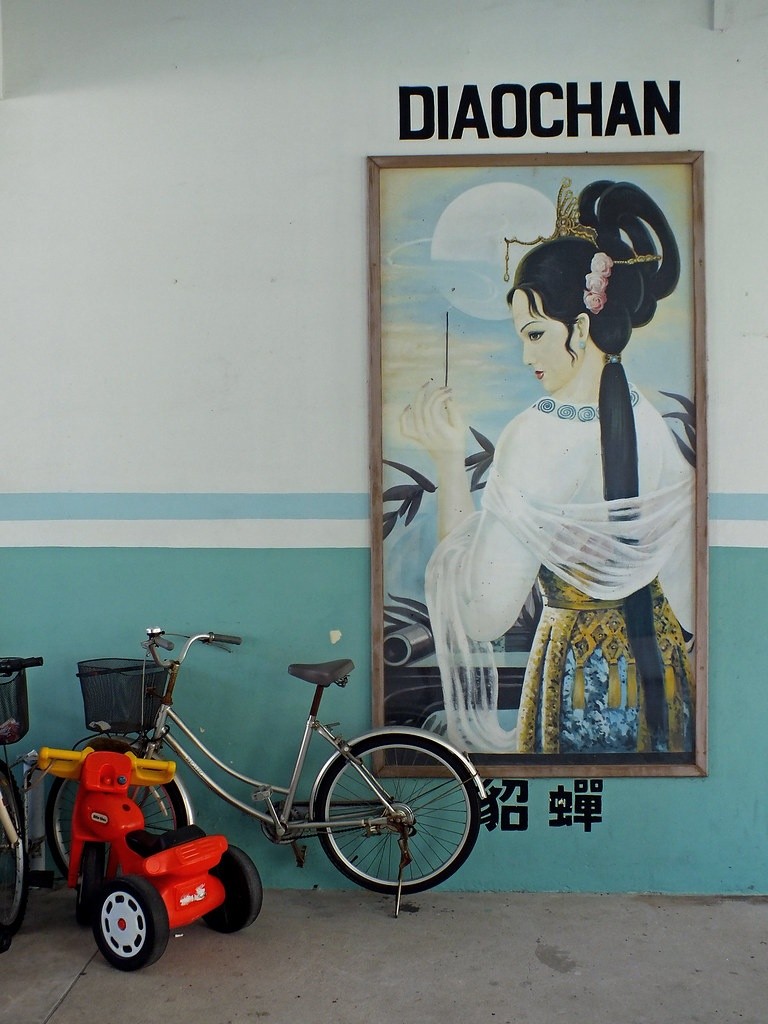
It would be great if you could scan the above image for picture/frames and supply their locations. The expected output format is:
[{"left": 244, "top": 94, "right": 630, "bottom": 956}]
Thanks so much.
[{"left": 368, "top": 153, "right": 710, "bottom": 780}]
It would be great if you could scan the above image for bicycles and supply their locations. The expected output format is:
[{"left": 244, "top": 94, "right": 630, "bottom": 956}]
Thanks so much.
[
  {"left": 45, "top": 625, "right": 491, "bottom": 918},
  {"left": 0, "top": 656, "right": 43, "bottom": 955}
]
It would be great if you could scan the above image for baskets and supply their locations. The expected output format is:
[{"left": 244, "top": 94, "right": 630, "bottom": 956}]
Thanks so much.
[
  {"left": 0, "top": 657, "right": 30, "bottom": 745},
  {"left": 76, "top": 657, "right": 170, "bottom": 734}
]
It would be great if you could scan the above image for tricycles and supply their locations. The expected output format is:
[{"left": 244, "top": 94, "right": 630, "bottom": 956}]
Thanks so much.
[{"left": 38, "top": 745, "right": 263, "bottom": 971}]
